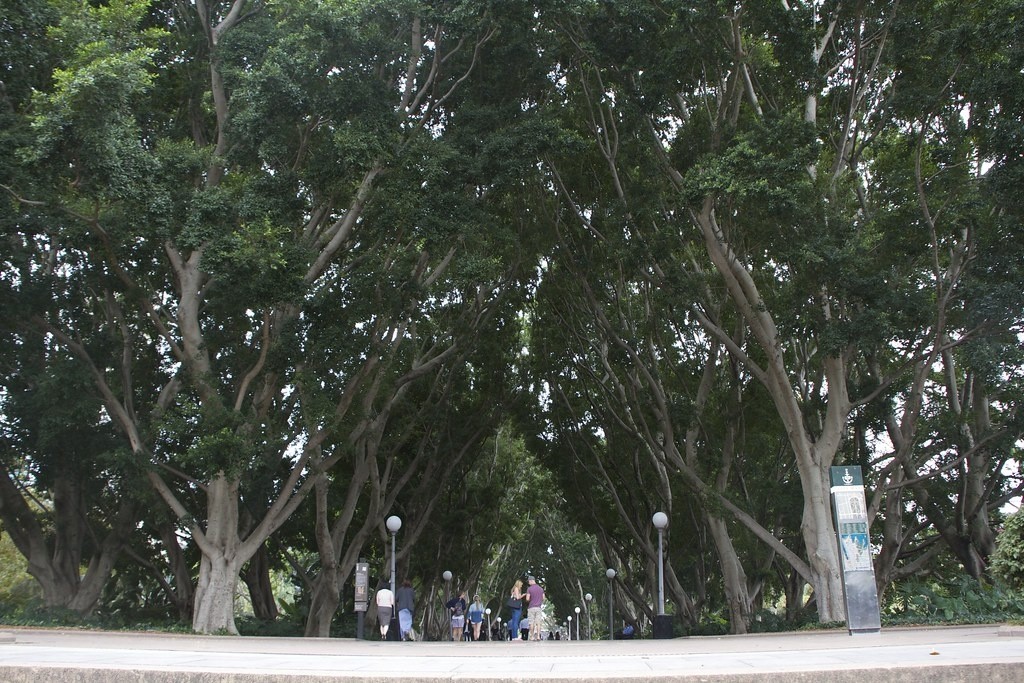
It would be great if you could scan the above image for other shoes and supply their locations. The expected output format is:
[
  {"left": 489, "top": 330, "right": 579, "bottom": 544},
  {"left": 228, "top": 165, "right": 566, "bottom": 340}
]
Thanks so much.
[
  {"left": 477, "top": 638, "right": 479, "bottom": 641},
  {"left": 512, "top": 638, "right": 523, "bottom": 641},
  {"left": 458, "top": 640, "right": 460, "bottom": 641},
  {"left": 403, "top": 638, "right": 406, "bottom": 642},
  {"left": 474, "top": 640, "right": 477, "bottom": 641},
  {"left": 452, "top": 636, "right": 454, "bottom": 641},
  {"left": 381, "top": 634, "right": 388, "bottom": 641},
  {"left": 530, "top": 639, "right": 534, "bottom": 641},
  {"left": 537, "top": 639, "right": 541, "bottom": 641}
]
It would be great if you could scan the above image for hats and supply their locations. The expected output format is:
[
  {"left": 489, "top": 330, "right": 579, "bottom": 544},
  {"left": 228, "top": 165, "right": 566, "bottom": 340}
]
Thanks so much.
[
  {"left": 457, "top": 590, "right": 463, "bottom": 598},
  {"left": 473, "top": 595, "right": 479, "bottom": 601},
  {"left": 528, "top": 576, "right": 535, "bottom": 580}
]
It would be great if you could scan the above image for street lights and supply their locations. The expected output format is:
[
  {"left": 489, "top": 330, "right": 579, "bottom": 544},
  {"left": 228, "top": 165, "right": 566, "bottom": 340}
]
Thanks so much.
[
  {"left": 503, "top": 623, "right": 507, "bottom": 642},
  {"left": 653, "top": 512, "right": 674, "bottom": 639},
  {"left": 585, "top": 593, "right": 592, "bottom": 640},
  {"left": 387, "top": 516, "right": 402, "bottom": 639},
  {"left": 485, "top": 608, "right": 491, "bottom": 633},
  {"left": 443, "top": 571, "right": 453, "bottom": 624},
  {"left": 567, "top": 616, "right": 572, "bottom": 640},
  {"left": 606, "top": 569, "right": 616, "bottom": 639},
  {"left": 497, "top": 617, "right": 501, "bottom": 640},
  {"left": 574, "top": 607, "right": 581, "bottom": 640}
]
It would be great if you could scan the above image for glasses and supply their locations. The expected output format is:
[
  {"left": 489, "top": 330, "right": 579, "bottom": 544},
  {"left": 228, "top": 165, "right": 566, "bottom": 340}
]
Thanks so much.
[{"left": 521, "top": 585, "right": 523, "bottom": 586}]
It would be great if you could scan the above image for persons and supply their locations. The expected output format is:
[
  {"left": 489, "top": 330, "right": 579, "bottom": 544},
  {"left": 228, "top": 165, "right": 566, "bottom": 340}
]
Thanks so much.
[
  {"left": 468, "top": 595, "right": 484, "bottom": 640},
  {"left": 520, "top": 614, "right": 530, "bottom": 641},
  {"left": 511, "top": 579, "right": 527, "bottom": 641},
  {"left": 526, "top": 576, "right": 545, "bottom": 642},
  {"left": 492, "top": 623, "right": 500, "bottom": 640},
  {"left": 446, "top": 590, "right": 467, "bottom": 641},
  {"left": 615, "top": 622, "right": 636, "bottom": 639},
  {"left": 508, "top": 614, "right": 512, "bottom": 640},
  {"left": 396, "top": 579, "right": 416, "bottom": 641},
  {"left": 463, "top": 618, "right": 486, "bottom": 642},
  {"left": 548, "top": 630, "right": 560, "bottom": 640},
  {"left": 376, "top": 583, "right": 395, "bottom": 640}
]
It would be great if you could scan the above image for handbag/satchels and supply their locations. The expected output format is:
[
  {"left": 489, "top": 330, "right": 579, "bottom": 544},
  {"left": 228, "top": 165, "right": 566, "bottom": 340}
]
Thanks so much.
[
  {"left": 451, "top": 608, "right": 457, "bottom": 616},
  {"left": 506, "top": 597, "right": 522, "bottom": 609}
]
